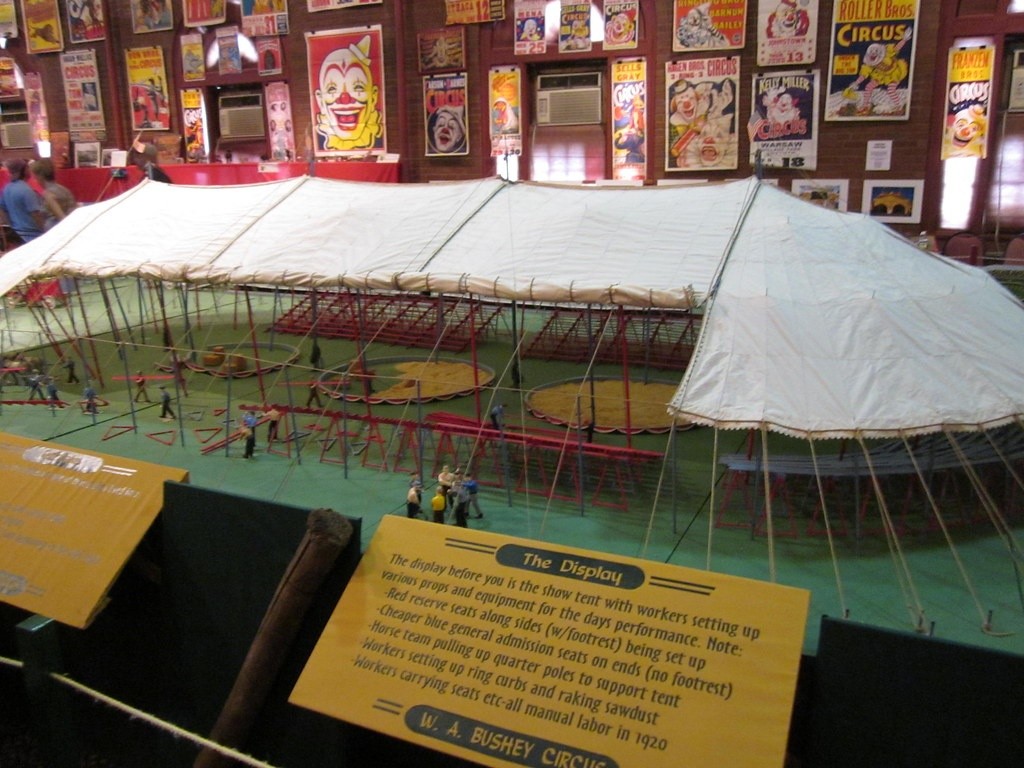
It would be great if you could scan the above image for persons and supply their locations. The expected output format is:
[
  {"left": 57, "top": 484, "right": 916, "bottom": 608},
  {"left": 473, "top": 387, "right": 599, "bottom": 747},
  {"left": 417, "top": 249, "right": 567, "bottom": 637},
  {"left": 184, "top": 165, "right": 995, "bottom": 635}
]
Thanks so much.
[
  {"left": 261, "top": 404, "right": 281, "bottom": 442},
  {"left": 12, "top": 352, "right": 79, "bottom": 409},
  {"left": 242, "top": 412, "right": 255, "bottom": 458},
  {"left": 446, "top": 474, "right": 483, "bottom": 527},
  {"left": 306, "top": 379, "right": 324, "bottom": 408},
  {"left": 84, "top": 380, "right": 99, "bottom": 413},
  {"left": 490, "top": 403, "right": 509, "bottom": 430},
  {"left": 133, "top": 142, "right": 173, "bottom": 181},
  {"left": 31, "top": 159, "right": 75, "bottom": 293},
  {"left": 158, "top": 387, "right": 176, "bottom": 419},
  {"left": 431, "top": 486, "right": 445, "bottom": 523},
  {"left": 0, "top": 158, "right": 44, "bottom": 242},
  {"left": 406, "top": 472, "right": 422, "bottom": 518},
  {"left": 134, "top": 371, "right": 151, "bottom": 401},
  {"left": 438, "top": 466, "right": 454, "bottom": 509}
]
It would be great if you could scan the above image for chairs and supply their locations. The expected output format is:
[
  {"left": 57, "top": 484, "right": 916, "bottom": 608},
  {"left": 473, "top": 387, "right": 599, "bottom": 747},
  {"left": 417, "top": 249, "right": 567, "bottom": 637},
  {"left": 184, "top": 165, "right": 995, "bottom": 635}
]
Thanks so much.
[
  {"left": 1004, "top": 232, "right": 1024, "bottom": 264},
  {"left": 943, "top": 229, "right": 986, "bottom": 265}
]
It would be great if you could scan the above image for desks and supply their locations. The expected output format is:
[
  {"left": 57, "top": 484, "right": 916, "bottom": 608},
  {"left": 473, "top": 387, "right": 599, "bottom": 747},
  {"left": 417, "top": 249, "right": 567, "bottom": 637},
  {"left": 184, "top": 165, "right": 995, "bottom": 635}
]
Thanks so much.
[{"left": 0, "top": 162, "right": 403, "bottom": 203}]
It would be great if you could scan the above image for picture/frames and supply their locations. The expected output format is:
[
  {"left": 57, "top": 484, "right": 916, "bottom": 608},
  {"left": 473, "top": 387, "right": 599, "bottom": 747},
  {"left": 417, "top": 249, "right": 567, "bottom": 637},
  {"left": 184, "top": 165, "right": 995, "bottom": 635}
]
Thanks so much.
[
  {"left": 789, "top": 178, "right": 849, "bottom": 210},
  {"left": 860, "top": 178, "right": 925, "bottom": 225}
]
